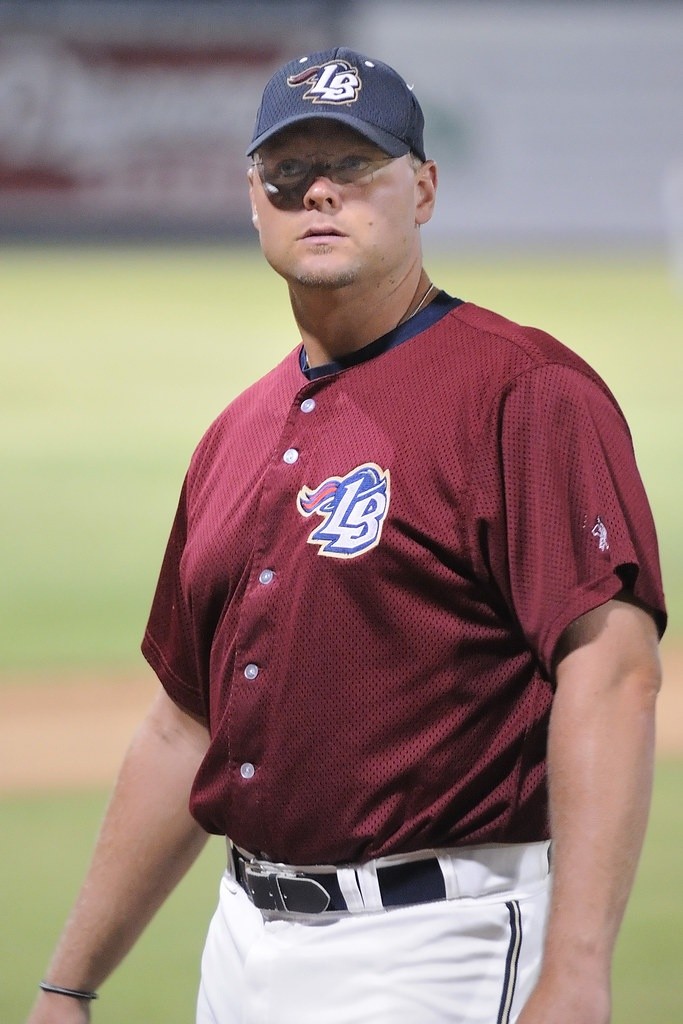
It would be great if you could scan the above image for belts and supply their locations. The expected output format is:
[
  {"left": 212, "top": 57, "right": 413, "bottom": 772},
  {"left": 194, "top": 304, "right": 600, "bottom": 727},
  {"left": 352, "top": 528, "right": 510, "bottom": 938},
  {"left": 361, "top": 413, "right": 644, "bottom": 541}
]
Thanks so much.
[{"left": 229, "top": 844, "right": 446, "bottom": 912}]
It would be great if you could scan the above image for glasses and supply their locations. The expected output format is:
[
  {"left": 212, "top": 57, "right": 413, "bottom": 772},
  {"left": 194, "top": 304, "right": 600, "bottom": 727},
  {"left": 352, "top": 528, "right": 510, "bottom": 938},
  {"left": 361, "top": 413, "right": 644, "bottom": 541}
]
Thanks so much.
[{"left": 252, "top": 150, "right": 412, "bottom": 197}]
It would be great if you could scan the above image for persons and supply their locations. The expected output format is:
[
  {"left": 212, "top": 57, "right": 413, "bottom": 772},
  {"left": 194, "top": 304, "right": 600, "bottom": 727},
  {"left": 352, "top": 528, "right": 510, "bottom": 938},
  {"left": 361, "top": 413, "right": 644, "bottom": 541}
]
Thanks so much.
[{"left": 31, "top": 48, "right": 671, "bottom": 1024}]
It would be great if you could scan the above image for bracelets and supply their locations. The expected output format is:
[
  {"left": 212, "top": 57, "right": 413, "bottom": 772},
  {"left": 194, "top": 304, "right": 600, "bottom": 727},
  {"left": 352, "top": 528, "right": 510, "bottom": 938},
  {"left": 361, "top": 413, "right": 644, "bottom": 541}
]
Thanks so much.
[{"left": 38, "top": 982, "right": 99, "bottom": 1000}]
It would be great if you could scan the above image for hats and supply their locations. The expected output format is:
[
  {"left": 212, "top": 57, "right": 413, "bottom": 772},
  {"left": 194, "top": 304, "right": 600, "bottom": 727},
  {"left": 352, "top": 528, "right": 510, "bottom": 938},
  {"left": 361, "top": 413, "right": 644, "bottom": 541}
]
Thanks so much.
[{"left": 246, "top": 47, "right": 427, "bottom": 173}]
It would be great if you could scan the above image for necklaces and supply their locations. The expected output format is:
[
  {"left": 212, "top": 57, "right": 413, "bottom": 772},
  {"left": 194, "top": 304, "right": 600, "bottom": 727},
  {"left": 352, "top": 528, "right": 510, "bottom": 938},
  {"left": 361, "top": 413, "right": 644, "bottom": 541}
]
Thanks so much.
[{"left": 305, "top": 283, "right": 434, "bottom": 371}]
